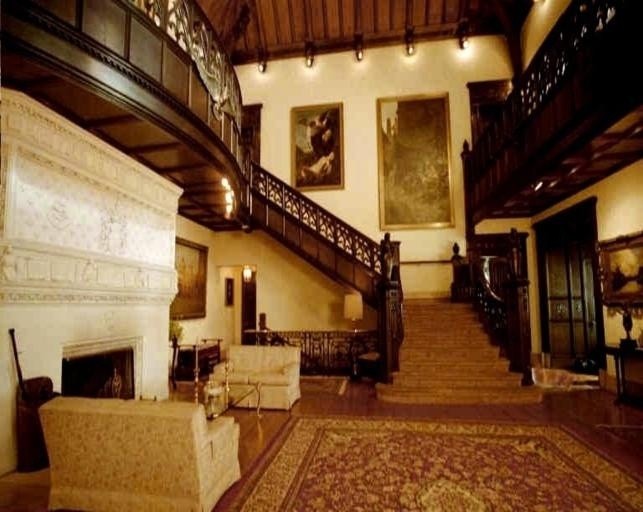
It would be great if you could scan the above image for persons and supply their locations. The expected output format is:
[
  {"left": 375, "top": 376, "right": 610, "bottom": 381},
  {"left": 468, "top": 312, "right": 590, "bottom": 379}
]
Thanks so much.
[{"left": 303, "top": 115, "right": 335, "bottom": 182}]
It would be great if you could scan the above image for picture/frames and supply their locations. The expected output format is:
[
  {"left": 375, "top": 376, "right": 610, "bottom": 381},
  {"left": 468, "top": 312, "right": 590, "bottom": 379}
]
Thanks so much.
[
  {"left": 374, "top": 90, "right": 456, "bottom": 233},
  {"left": 170, "top": 234, "right": 209, "bottom": 320},
  {"left": 595, "top": 228, "right": 643, "bottom": 310},
  {"left": 289, "top": 102, "right": 344, "bottom": 193}
]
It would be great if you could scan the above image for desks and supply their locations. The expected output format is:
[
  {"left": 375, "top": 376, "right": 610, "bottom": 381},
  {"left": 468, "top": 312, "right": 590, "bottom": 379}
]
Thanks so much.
[
  {"left": 603, "top": 343, "right": 643, "bottom": 406},
  {"left": 344, "top": 329, "right": 370, "bottom": 377}
]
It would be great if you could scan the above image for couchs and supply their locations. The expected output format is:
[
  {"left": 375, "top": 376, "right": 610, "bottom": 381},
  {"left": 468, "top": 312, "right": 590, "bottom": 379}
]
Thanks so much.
[
  {"left": 36, "top": 392, "right": 244, "bottom": 511},
  {"left": 217, "top": 343, "right": 302, "bottom": 411}
]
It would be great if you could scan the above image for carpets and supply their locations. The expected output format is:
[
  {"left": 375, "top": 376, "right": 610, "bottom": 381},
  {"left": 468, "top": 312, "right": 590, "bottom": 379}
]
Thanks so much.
[{"left": 212, "top": 413, "right": 643, "bottom": 511}]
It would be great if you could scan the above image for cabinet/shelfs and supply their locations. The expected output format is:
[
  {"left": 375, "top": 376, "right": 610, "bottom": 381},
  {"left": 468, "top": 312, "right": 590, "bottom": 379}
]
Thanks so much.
[{"left": 174, "top": 335, "right": 224, "bottom": 378}]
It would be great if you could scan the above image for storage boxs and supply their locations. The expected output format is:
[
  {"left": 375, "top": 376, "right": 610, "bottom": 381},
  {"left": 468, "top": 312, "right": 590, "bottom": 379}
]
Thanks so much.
[{"left": 358, "top": 357, "right": 381, "bottom": 378}]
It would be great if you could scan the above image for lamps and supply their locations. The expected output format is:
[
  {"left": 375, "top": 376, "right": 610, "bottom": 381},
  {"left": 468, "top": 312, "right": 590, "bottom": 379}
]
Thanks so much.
[
  {"left": 342, "top": 290, "right": 364, "bottom": 331},
  {"left": 256, "top": 36, "right": 473, "bottom": 75},
  {"left": 242, "top": 264, "right": 252, "bottom": 283}
]
